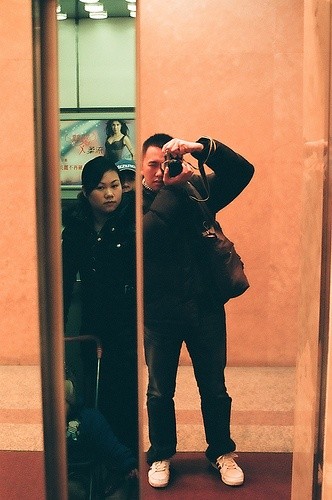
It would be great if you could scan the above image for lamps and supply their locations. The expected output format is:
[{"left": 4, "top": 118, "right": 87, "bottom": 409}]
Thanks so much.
[{"left": 56, "top": 0, "right": 136, "bottom": 21}]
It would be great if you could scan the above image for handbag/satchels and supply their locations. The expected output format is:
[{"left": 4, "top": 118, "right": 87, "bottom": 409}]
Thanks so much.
[{"left": 185, "top": 181, "right": 249, "bottom": 304}]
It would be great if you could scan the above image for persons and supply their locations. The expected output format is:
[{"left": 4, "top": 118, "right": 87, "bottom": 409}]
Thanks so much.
[
  {"left": 64, "top": 371, "right": 140, "bottom": 500},
  {"left": 61, "top": 158, "right": 136, "bottom": 461},
  {"left": 103, "top": 119, "right": 133, "bottom": 163},
  {"left": 141, "top": 133, "right": 255, "bottom": 491}
]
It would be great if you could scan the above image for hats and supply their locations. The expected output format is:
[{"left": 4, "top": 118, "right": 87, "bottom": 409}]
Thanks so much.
[{"left": 114, "top": 159, "right": 136, "bottom": 173}]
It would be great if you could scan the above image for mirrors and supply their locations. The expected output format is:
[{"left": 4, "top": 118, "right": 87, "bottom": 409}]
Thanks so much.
[{"left": 1, "top": 0, "right": 331, "bottom": 500}]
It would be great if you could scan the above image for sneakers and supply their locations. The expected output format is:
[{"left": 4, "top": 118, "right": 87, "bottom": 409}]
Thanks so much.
[
  {"left": 210, "top": 451, "right": 244, "bottom": 486},
  {"left": 148, "top": 459, "right": 170, "bottom": 487}
]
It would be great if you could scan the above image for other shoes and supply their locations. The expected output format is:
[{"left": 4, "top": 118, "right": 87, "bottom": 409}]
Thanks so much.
[{"left": 104, "top": 474, "right": 125, "bottom": 497}]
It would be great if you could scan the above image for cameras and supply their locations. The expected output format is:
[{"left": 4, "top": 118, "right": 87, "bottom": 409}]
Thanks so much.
[{"left": 160, "top": 150, "right": 184, "bottom": 177}]
